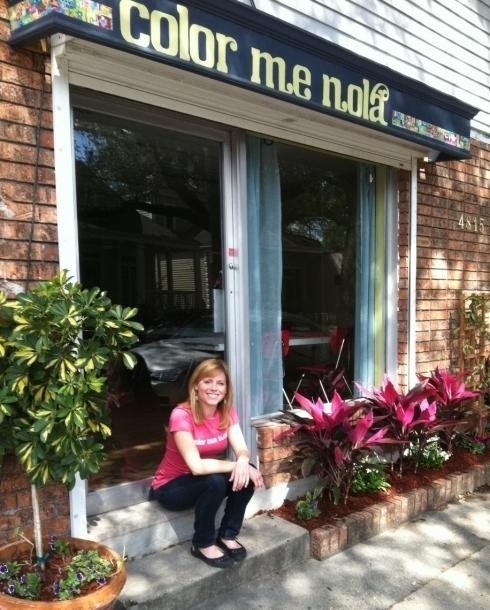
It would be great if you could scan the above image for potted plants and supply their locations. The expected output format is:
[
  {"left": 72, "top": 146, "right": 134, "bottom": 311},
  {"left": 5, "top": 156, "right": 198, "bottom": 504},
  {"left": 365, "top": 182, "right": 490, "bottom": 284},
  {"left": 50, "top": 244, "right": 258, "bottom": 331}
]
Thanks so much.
[{"left": 0, "top": 268, "right": 146, "bottom": 610}]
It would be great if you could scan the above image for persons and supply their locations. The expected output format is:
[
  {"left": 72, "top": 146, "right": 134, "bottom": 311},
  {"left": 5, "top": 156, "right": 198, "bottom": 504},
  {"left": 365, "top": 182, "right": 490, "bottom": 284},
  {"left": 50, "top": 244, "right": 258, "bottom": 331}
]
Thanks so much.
[{"left": 149, "top": 358, "right": 264, "bottom": 569}]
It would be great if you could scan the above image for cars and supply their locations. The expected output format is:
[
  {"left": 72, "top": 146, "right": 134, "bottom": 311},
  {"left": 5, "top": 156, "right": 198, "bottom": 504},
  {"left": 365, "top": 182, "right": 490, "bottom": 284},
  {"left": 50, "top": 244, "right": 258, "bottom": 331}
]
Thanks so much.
[{"left": 125, "top": 310, "right": 334, "bottom": 407}]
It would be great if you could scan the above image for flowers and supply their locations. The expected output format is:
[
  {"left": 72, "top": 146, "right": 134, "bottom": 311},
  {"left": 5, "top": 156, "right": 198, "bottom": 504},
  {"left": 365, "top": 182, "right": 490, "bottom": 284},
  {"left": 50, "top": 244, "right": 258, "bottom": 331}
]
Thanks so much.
[
  {"left": 277, "top": 368, "right": 483, "bottom": 499},
  {"left": 0, "top": 535, "right": 113, "bottom": 601}
]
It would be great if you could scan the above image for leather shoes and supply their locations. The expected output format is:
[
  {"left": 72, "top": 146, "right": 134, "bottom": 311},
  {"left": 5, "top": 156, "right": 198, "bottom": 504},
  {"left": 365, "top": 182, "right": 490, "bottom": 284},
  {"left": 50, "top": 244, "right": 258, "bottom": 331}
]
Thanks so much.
[
  {"left": 216, "top": 536, "right": 246, "bottom": 561},
  {"left": 191, "top": 543, "right": 235, "bottom": 569}
]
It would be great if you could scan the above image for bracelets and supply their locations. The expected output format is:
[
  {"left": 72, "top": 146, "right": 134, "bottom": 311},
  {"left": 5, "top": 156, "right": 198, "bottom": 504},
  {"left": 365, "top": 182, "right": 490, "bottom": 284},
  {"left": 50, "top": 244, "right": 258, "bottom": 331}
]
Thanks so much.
[{"left": 235, "top": 449, "right": 252, "bottom": 459}]
